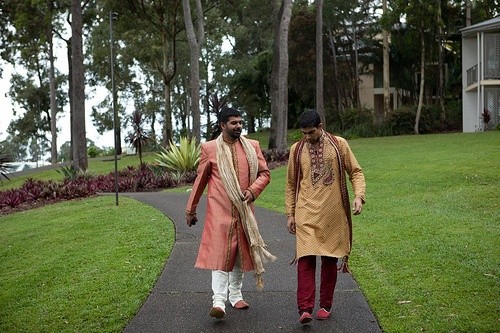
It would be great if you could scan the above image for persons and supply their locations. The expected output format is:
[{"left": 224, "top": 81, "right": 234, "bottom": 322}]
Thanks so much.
[
  {"left": 185, "top": 107, "right": 271, "bottom": 318},
  {"left": 284, "top": 110, "right": 366, "bottom": 324}
]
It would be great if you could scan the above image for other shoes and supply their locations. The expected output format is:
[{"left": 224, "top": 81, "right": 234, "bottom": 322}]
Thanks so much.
[
  {"left": 315, "top": 308, "right": 331, "bottom": 320},
  {"left": 299, "top": 311, "right": 314, "bottom": 324},
  {"left": 234, "top": 300, "right": 249, "bottom": 309},
  {"left": 209, "top": 307, "right": 225, "bottom": 319}
]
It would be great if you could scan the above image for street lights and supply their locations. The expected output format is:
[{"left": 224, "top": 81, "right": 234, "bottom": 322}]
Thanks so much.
[{"left": 109, "top": 9, "right": 119, "bottom": 205}]
